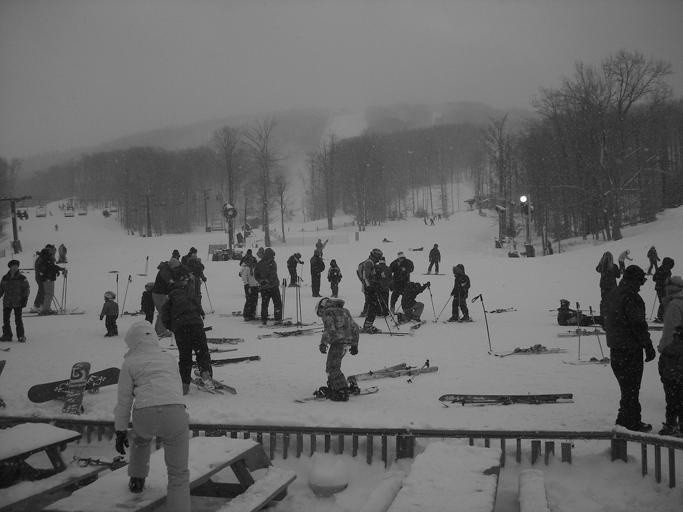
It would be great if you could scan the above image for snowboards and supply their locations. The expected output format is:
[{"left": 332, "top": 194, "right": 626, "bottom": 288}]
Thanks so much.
[
  {"left": 62, "top": 361, "right": 91, "bottom": 413},
  {"left": 28, "top": 367, "right": 119, "bottom": 402}
]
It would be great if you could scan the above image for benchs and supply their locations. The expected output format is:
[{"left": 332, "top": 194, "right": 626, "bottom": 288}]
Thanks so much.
[
  {"left": 0, "top": 459, "right": 110, "bottom": 512},
  {"left": 217, "top": 468, "right": 297, "bottom": 512},
  {"left": 516, "top": 468, "right": 550, "bottom": 512},
  {"left": 359, "top": 469, "right": 406, "bottom": 511}
]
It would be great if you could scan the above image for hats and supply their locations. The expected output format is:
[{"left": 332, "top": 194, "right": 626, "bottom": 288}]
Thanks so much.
[
  {"left": 168, "top": 257, "right": 181, "bottom": 270},
  {"left": 314, "top": 296, "right": 330, "bottom": 317},
  {"left": 144, "top": 282, "right": 155, "bottom": 291},
  {"left": 103, "top": 291, "right": 116, "bottom": 300},
  {"left": 560, "top": 299, "right": 570, "bottom": 307},
  {"left": 7, "top": 259, "right": 21, "bottom": 268},
  {"left": 665, "top": 276, "right": 683, "bottom": 292},
  {"left": 369, "top": 248, "right": 383, "bottom": 258}
]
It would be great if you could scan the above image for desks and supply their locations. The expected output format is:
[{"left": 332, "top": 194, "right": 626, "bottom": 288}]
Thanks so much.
[
  {"left": 42, "top": 437, "right": 260, "bottom": 512},
  {"left": 387, "top": 445, "right": 502, "bottom": 511},
  {"left": 0, "top": 423, "right": 84, "bottom": 474}
]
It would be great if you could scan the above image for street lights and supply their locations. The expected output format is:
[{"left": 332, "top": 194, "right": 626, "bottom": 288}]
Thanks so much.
[{"left": 518, "top": 192, "right": 531, "bottom": 239}]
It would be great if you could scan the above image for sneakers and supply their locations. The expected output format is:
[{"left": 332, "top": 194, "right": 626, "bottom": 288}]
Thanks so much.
[
  {"left": 104, "top": 332, "right": 118, "bottom": 337},
  {"left": 448, "top": 315, "right": 459, "bottom": 322},
  {"left": 657, "top": 421, "right": 683, "bottom": 438},
  {"left": 242, "top": 314, "right": 283, "bottom": 325},
  {"left": 458, "top": 315, "right": 469, "bottom": 321},
  {"left": 127, "top": 476, "right": 145, "bottom": 493},
  {"left": 29, "top": 305, "right": 56, "bottom": 315},
  {"left": 17, "top": 337, "right": 26, "bottom": 342},
  {"left": 157, "top": 330, "right": 172, "bottom": 337},
  {"left": 311, "top": 294, "right": 338, "bottom": 298},
  {"left": 0, "top": 335, "right": 12, "bottom": 341},
  {"left": 327, "top": 388, "right": 349, "bottom": 401},
  {"left": 615, "top": 418, "right": 652, "bottom": 433},
  {"left": 360, "top": 307, "right": 422, "bottom": 334}
]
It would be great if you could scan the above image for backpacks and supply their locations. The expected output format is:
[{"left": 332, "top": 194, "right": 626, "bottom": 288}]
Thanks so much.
[{"left": 355, "top": 258, "right": 376, "bottom": 283}]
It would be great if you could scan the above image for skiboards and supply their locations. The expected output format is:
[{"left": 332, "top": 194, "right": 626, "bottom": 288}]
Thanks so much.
[
  {"left": 296, "top": 387, "right": 378, "bottom": 402},
  {"left": 496, "top": 347, "right": 560, "bottom": 354},
  {"left": 439, "top": 394, "right": 573, "bottom": 405},
  {"left": 194, "top": 323, "right": 259, "bottom": 394},
  {"left": 376, "top": 332, "right": 409, "bottom": 337},
  {"left": 21, "top": 308, "right": 84, "bottom": 317},
  {"left": 349, "top": 363, "right": 438, "bottom": 380},
  {"left": 223, "top": 313, "right": 321, "bottom": 339},
  {"left": 562, "top": 360, "right": 612, "bottom": 365},
  {"left": 560, "top": 331, "right": 605, "bottom": 338}
]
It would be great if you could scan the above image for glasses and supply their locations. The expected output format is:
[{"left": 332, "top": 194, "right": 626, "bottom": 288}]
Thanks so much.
[
  {"left": 640, "top": 277, "right": 647, "bottom": 285},
  {"left": 663, "top": 277, "right": 682, "bottom": 289}
]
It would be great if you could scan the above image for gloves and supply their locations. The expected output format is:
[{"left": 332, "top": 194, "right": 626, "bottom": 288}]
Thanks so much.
[
  {"left": 348, "top": 344, "right": 358, "bottom": 355},
  {"left": 318, "top": 343, "right": 327, "bottom": 354},
  {"left": 643, "top": 345, "right": 656, "bottom": 362},
  {"left": 55, "top": 271, "right": 60, "bottom": 277},
  {"left": 114, "top": 430, "right": 129, "bottom": 454}
]
